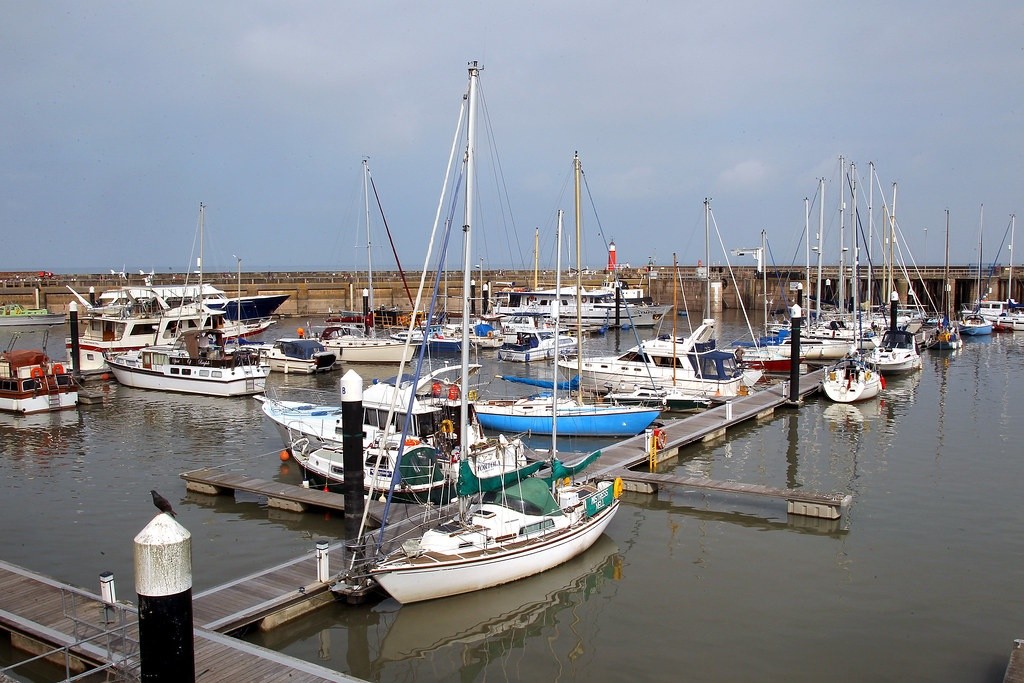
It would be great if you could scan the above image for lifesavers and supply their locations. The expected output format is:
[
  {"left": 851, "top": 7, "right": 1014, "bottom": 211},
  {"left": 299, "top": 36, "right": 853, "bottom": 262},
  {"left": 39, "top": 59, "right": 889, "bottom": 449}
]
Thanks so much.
[
  {"left": 488, "top": 331, "right": 492, "bottom": 337},
  {"left": 945, "top": 333, "right": 951, "bottom": 341},
  {"left": 581, "top": 297, "right": 587, "bottom": 303},
  {"left": 52, "top": 365, "right": 64, "bottom": 375},
  {"left": 613, "top": 477, "right": 623, "bottom": 499},
  {"left": 405, "top": 440, "right": 420, "bottom": 446},
  {"left": 441, "top": 419, "right": 453, "bottom": 433},
  {"left": 865, "top": 371, "right": 870, "bottom": 381},
  {"left": 657, "top": 430, "right": 667, "bottom": 449},
  {"left": 31, "top": 368, "right": 45, "bottom": 379},
  {"left": 15, "top": 309, "right": 21, "bottom": 314},
  {"left": 469, "top": 390, "right": 477, "bottom": 401}
]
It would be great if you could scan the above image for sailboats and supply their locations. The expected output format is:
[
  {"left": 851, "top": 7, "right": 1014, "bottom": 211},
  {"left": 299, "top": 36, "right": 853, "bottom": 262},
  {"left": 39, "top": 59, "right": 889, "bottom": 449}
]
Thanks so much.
[
  {"left": 102, "top": 200, "right": 272, "bottom": 398},
  {"left": 297, "top": 157, "right": 430, "bottom": 363},
  {"left": 957, "top": 202, "right": 995, "bottom": 337},
  {"left": 864, "top": 182, "right": 923, "bottom": 376},
  {"left": 815, "top": 161, "right": 885, "bottom": 403},
  {"left": 472, "top": 149, "right": 670, "bottom": 439},
  {"left": 924, "top": 209, "right": 964, "bottom": 351},
  {"left": 387, "top": 149, "right": 675, "bottom": 362},
  {"left": 328, "top": 60, "right": 622, "bottom": 606},
  {"left": 690, "top": 151, "right": 944, "bottom": 392}
]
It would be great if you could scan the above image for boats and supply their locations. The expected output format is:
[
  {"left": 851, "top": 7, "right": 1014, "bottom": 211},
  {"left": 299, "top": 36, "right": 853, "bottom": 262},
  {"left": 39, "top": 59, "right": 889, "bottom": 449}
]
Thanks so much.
[
  {"left": 554, "top": 316, "right": 754, "bottom": 412},
  {"left": 252, "top": 362, "right": 531, "bottom": 488},
  {"left": 497, "top": 315, "right": 578, "bottom": 362},
  {"left": 241, "top": 337, "right": 337, "bottom": 374},
  {"left": 0, "top": 328, "right": 80, "bottom": 415},
  {"left": 0, "top": 303, "right": 48, "bottom": 316},
  {"left": 288, "top": 425, "right": 454, "bottom": 506},
  {"left": 65, "top": 280, "right": 291, "bottom": 374},
  {"left": 958, "top": 213, "right": 1024, "bottom": 332}
]
